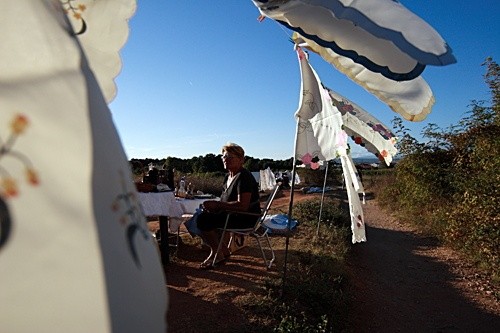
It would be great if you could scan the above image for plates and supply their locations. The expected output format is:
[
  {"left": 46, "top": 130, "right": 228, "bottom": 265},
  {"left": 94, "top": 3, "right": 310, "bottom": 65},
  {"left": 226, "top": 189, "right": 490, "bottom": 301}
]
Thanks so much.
[{"left": 194, "top": 195, "right": 212, "bottom": 198}]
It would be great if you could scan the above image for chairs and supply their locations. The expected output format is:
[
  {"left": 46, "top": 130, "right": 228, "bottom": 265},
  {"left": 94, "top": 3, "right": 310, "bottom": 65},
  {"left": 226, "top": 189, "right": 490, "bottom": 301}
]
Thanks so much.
[{"left": 197, "top": 181, "right": 282, "bottom": 270}]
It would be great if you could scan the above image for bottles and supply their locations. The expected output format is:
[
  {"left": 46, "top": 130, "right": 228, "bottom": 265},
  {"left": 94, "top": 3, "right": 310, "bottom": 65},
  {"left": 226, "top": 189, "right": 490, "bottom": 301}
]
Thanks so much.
[
  {"left": 187, "top": 183, "right": 195, "bottom": 199},
  {"left": 178, "top": 177, "right": 186, "bottom": 198}
]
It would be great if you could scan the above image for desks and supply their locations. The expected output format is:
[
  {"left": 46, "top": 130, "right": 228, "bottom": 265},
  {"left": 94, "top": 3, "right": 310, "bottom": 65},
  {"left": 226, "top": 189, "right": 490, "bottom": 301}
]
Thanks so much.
[
  {"left": 137, "top": 191, "right": 179, "bottom": 272},
  {"left": 173, "top": 194, "right": 221, "bottom": 215}
]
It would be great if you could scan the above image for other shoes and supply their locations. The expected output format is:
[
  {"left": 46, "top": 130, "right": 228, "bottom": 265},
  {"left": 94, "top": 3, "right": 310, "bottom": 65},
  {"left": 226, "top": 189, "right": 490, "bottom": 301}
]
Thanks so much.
[{"left": 200, "top": 249, "right": 230, "bottom": 268}]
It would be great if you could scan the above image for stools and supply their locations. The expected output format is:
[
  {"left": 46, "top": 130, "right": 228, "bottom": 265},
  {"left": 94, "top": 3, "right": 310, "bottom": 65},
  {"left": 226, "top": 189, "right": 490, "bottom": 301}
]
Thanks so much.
[{"left": 165, "top": 216, "right": 194, "bottom": 265}]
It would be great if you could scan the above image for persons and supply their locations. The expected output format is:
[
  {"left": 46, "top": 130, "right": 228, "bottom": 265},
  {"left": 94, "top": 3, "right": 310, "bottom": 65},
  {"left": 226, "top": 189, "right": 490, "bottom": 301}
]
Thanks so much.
[{"left": 196, "top": 143, "right": 262, "bottom": 268}]
